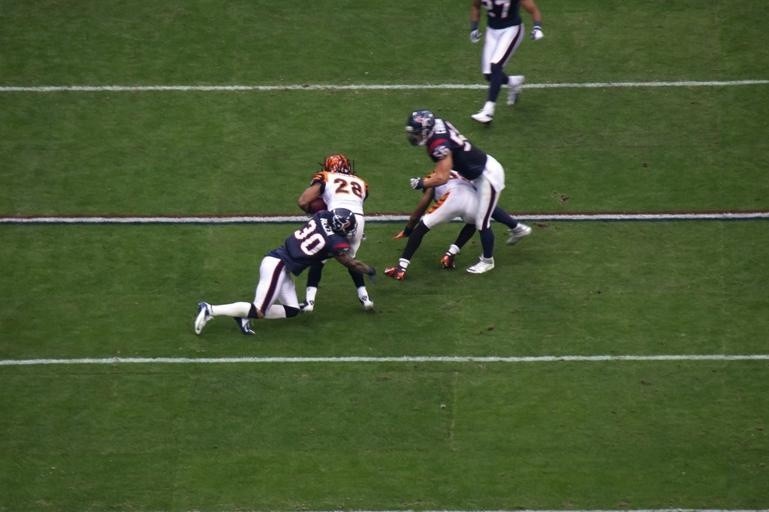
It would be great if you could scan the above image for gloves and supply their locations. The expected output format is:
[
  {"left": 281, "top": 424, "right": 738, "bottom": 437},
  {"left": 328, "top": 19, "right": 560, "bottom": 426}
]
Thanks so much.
[
  {"left": 529, "top": 25, "right": 544, "bottom": 41},
  {"left": 470, "top": 109, "right": 496, "bottom": 124},
  {"left": 470, "top": 28, "right": 483, "bottom": 43},
  {"left": 410, "top": 176, "right": 424, "bottom": 190}
]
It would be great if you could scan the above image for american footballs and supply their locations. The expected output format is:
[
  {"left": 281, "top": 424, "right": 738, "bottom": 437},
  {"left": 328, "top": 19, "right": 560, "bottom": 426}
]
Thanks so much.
[{"left": 309, "top": 199, "right": 328, "bottom": 213}]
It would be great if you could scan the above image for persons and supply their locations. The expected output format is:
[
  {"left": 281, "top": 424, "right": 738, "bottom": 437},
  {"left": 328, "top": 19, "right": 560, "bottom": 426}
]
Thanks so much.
[
  {"left": 383, "top": 169, "right": 479, "bottom": 281},
  {"left": 467, "top": 0, "right": 544, "bottom": 124},
  {"left": 297, "top": 154, "right": 375, "bottom": 314},
  {"left": 404, "top": 109, "right": 532, "bottom": 275},
  {"left": 194, "top": 207, "right": 379, "bottom": 336}
]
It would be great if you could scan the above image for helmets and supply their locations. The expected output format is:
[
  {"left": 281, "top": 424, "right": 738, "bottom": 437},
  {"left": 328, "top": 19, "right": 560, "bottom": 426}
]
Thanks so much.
[
  {"left": 323, "top": 153, "right": 352, "bottom": 174},
  {"left": 404, "top": 108, "right": 437, "bottom": 148},
  {"left": 327, "top": 207, "right": 358, "bottom": 239}
]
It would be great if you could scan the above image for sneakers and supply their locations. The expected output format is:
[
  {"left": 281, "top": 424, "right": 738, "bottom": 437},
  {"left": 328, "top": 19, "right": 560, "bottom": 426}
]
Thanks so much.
[
  {"left": 232, "top": 315, "right": 257, "bottom": 337},
  {"left": 294, "top": 298, "right": 315, "bottom": 313},
  {"left": 357, "top": 295, "right": 378, "bottom": 311},
  {"left": 193, "top": 301, "right": 213, "bottom": 336},
  {"left": 465, "top": 254, "right": 496, "bottom": 275},
  {"left": 505, "top": 221, "right": 533, "bottom": 247},
  {"left": 507, "top": 75, "right": 525, "bottom": 107},
  {"left": 383, "top": 266, "right": 408, "bottom": 282},
  {"left": 439, "top": 251, "right": 458, "bottom": 272}
]
